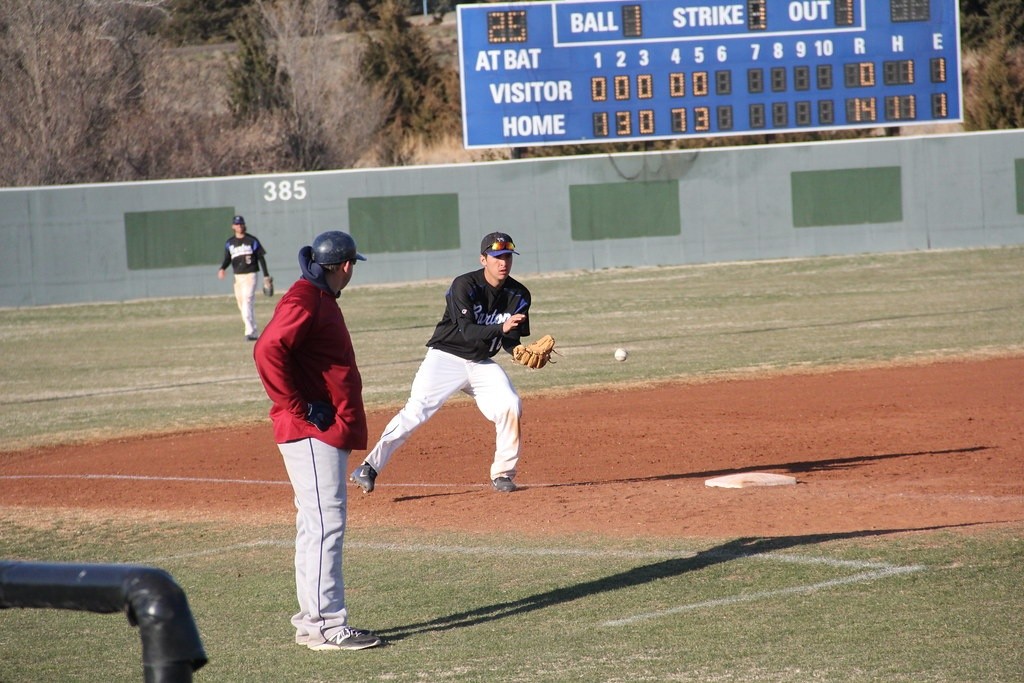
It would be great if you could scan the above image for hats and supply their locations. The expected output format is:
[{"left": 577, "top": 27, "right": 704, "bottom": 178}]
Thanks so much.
[
  {"left": 481, "top": 232, "right": 519, "bottom": 256},
  {"left": 233, "top": 216, "right": 245, "bottom": 224}
]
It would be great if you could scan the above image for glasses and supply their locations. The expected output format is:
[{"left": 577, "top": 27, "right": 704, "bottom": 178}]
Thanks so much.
[{"left": 481, "top": 242, "right": 515, "bottom": 254}]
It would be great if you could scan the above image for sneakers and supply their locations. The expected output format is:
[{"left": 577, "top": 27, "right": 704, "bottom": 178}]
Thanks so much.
[
  {"left": 298, "top": 627, "right": 372, "bottom": 645},
  {"left": 492, "top": 477, "right": 516, "bottom": 491},
  {"left": 349, "top": 462, "right": 377, "bottom": 493},
  {"left": 310, "top": 626, "right": 381, "bottom": 650}
]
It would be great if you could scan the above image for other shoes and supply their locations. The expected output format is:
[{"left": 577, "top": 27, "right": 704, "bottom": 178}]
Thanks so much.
[{"left": 244, "top": 335, "right": 257, "bottom": 341}]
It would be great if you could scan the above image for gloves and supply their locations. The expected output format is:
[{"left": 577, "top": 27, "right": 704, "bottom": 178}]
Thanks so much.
[{"left": 306, "top": 400, "right": 333, "bottom": 431}]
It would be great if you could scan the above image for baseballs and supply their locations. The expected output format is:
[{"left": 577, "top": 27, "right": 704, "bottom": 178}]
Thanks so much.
[{"left": 614, "top": 348, "right": 628, "bottom": 362}]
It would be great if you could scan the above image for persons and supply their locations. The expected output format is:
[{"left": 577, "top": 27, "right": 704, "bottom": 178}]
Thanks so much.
[
  {"left": 349, "top": 232, "right": 555, "bottom": 494},
  {"left": 254, "top": 232, "right": 381, "bottom": 653},
  {"left": 218, "top": 215, "right": 273, "bottom": 342}
]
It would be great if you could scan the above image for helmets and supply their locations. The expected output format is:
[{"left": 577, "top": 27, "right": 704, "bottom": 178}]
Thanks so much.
[{"left": 311, "top": 230, "right": 367, "bottom": 265}]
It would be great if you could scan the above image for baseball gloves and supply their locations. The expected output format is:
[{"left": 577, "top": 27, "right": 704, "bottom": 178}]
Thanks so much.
[
  {"left": 510, "top": 334, "right": 564, "bottom": 371},
  {"left": 262, "top": 276, "right": 273, "bottom": 296}
]
